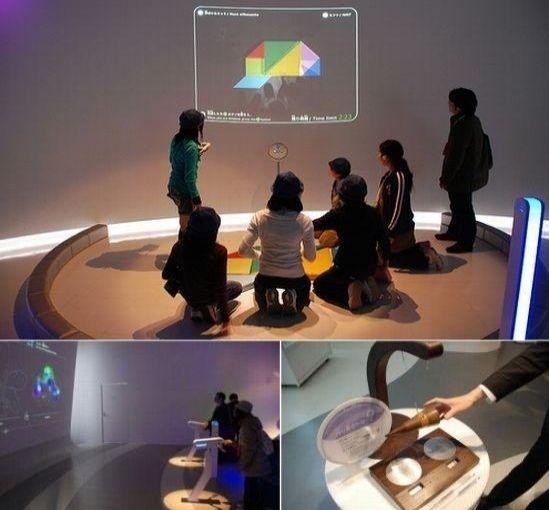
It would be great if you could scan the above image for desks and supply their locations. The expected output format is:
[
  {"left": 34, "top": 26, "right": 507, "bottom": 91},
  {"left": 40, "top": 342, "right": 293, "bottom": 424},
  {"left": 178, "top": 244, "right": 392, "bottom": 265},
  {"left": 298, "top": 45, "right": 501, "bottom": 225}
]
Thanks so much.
[{"left": 323, "top": 407, "right": 490, "bottom": 510}]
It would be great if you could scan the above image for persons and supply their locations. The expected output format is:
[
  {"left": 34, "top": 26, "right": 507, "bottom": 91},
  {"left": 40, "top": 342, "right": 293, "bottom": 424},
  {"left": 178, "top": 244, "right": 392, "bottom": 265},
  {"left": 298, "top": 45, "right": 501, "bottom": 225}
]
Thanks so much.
[
  {"left": 160, "top": 206, "right": 244, "bottom": 330},
  {"left": 200, "top": 391, "right": 278, "bottom": 509},
  {"left": 328, "top": 156, "right": 351, "bottom": 208},
  {"left": 241, "top": 172, "right": 316, "bottom": 316},
  {"left": 375, "top": 139, "right": 439, "bottom": 271},
  {"left": 425, "top": 340, "right": 549, "bottom": 508},
  {"left": 168, "top": 110, "right": 211, "bottom": 238},
  {"left": 312, "top": 175, "right": 389, "bottom": 310},
  {"left": 433, "top": 87, "right": 493, "bottom": 253}
]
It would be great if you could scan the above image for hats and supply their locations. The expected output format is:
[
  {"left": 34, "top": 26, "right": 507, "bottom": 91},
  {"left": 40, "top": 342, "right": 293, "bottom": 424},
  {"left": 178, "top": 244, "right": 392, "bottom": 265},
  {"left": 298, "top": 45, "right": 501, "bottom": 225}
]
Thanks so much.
[{"left": 236, "top": 402, "right": 252, "bottom": 412}]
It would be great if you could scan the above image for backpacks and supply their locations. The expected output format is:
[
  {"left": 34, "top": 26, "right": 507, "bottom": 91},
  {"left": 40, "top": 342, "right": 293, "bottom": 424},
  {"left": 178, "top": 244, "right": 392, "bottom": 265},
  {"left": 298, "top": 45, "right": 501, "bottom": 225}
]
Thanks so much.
[{"left": 253, "top": 426, "right": 274, "bottom": 455}]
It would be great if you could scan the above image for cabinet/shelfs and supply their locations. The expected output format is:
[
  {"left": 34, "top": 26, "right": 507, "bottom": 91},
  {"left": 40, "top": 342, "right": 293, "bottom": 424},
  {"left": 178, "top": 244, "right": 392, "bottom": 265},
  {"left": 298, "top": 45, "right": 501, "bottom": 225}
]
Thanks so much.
[{"left": 281, "top": 341, "right": 333, "bottom": 389}]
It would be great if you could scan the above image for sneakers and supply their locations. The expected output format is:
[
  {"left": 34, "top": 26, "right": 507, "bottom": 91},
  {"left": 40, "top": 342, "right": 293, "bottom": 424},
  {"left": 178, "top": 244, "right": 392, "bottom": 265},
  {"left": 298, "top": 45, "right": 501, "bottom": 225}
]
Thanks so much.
[{"left": 190, "top": 233, "right": 473, "bottom": 324}]
